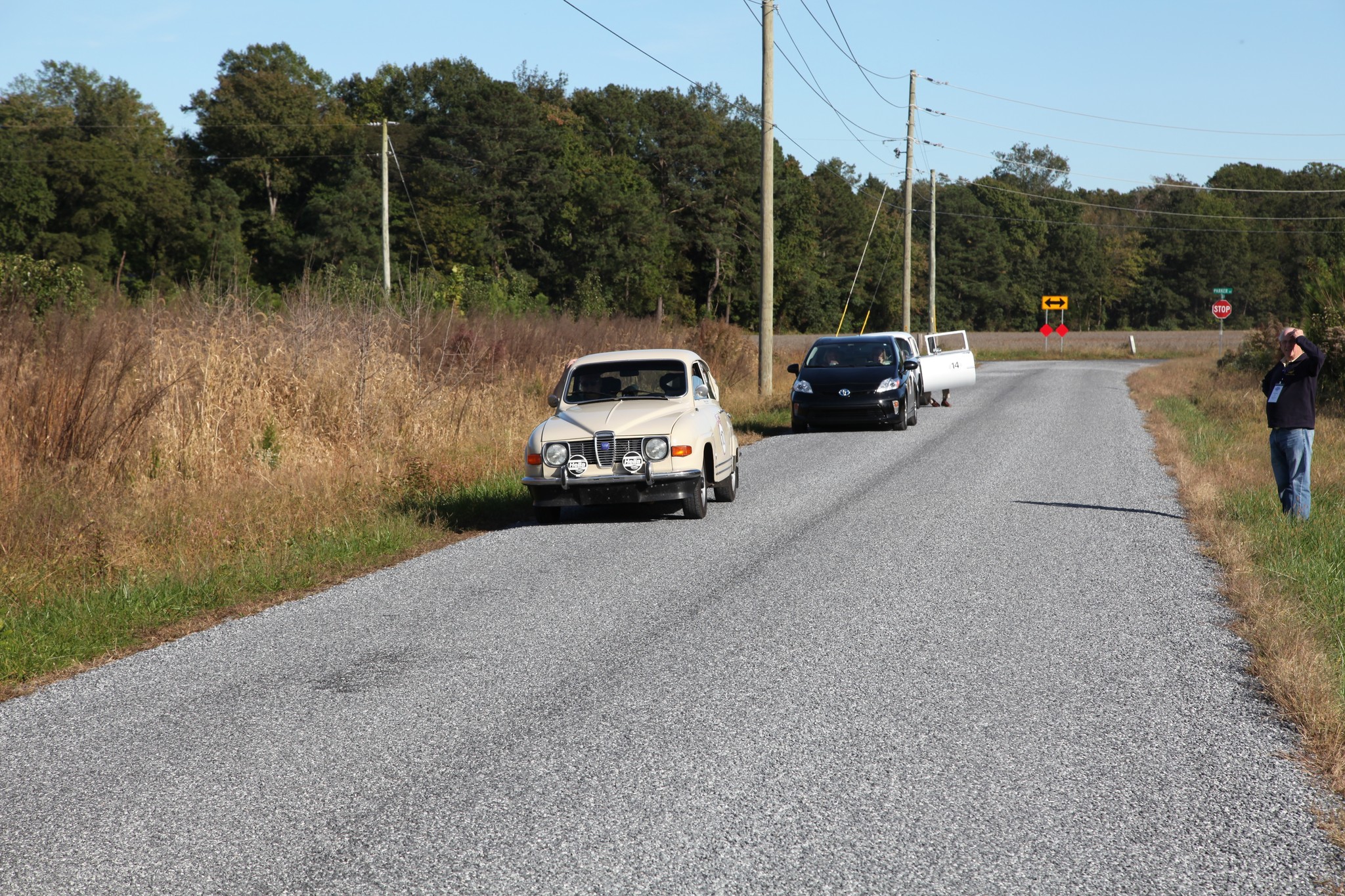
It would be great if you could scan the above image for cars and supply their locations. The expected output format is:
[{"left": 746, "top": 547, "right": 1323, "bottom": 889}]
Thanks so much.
[
  {"left": 787, "top": 335, "right": 918, "bottom": 433},
  {"left": 519, "top": 348, "right": 740, "bottom": 520},
  {"left": 848, "top": 330, "right": 976, "bottom": 408}
]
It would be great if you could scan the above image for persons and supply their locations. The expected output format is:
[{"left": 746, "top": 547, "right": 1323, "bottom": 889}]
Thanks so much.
[
  {"left": 870, "top": 347, "right": 890, "bottom": 365},
  {"left": 923, "top": 389, "right": 952, "bottom": 407},
  {"left": 825, "top": 349, "right": 841, "bottom": 365},
  {"left": 1262, "top": 327, "right": 1324, "bottom": 520},
  {"left": 566, "top": 372, "right": 616, "bottom": 402}
]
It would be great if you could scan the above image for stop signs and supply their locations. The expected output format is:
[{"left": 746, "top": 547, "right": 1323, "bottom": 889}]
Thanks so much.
[{"left": 1213, "top": 299, "right": 1231, "bottom": 319}]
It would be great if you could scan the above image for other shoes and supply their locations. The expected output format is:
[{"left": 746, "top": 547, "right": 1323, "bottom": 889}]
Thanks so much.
[
  {"left": 930, "top": 398, "right": 940, "bottom": 407},
  {"left": 941, "top": 400, "right": 951, "bottom": 407}
]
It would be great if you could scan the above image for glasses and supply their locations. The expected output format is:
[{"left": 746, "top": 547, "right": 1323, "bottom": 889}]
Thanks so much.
[
  {"left": 1280, "top": 339, "right": 1295, "bottom": 343},
  {"left": 581, "top": 381, "right": 596, "bottom": 387}
]
